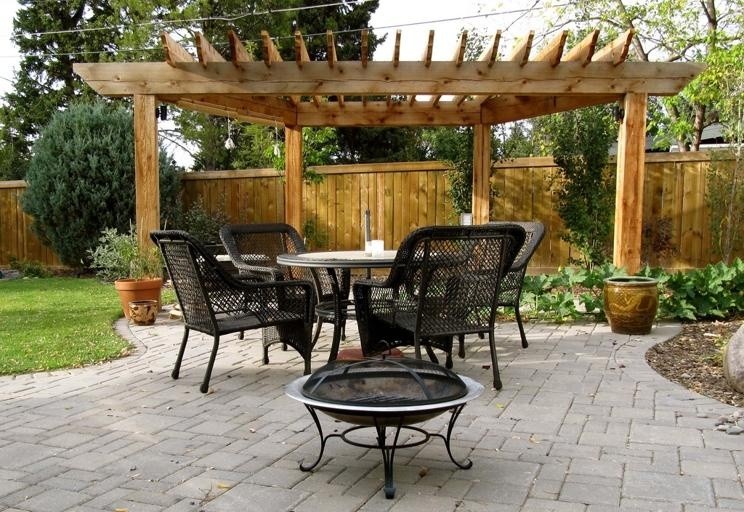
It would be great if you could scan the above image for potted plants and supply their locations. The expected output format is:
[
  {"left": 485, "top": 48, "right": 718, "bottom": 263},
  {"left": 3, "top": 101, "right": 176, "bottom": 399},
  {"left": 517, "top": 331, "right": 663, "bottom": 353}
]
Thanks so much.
[{"left": 85, "top": 217, "right": 168, "bottom": 319}]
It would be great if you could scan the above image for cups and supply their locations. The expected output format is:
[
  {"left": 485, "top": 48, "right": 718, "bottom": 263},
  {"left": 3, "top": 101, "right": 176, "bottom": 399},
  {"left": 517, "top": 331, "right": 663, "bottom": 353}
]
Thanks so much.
[{"left": 371, "top": 239, "right": 384, "bottom": 258}]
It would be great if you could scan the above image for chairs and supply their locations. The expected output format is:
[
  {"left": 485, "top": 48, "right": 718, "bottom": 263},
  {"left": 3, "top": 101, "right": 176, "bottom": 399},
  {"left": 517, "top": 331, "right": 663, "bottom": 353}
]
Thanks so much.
[
  {"left": 457, "top": 220, "right": 545, "bottom": 357},
  {"left": 148, "top": 230, "right": 315, "bottom": 395},
  {"left": 221, "top": 224, "right": 346, "bottom": 364},
  {"left": 352, "top": 225, "right": 526, "bottom": 389}
]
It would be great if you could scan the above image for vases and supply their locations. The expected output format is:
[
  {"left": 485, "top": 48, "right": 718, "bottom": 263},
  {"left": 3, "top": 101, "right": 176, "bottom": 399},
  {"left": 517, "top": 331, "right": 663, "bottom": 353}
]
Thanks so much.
[{"left": 130, "top": 300, "right": 159, "bottom": 326}]
